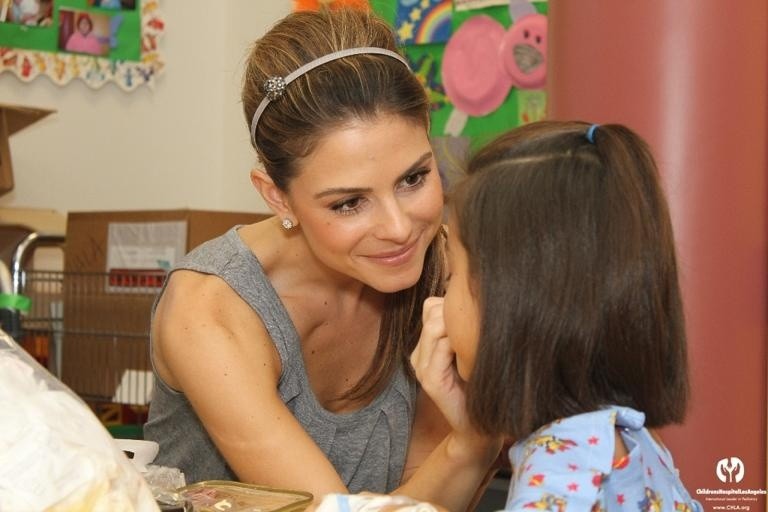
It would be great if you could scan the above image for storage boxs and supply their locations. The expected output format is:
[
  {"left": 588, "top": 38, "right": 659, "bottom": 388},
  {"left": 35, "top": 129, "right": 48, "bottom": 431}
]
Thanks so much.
[{"left": 60, "top": 209, "right": 276, "bottom": 397}]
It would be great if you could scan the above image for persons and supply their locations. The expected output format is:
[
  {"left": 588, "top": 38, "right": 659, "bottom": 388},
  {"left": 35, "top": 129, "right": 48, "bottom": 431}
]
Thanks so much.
[
  {"left": 64, "top": 14, "right": 101, "bottom": 54},
  {"left": 444, "top": 122, "right": 703, "bottom": 512},
  {"left": 142, "top": 11, "right": 510, "bottom": 512}
]
